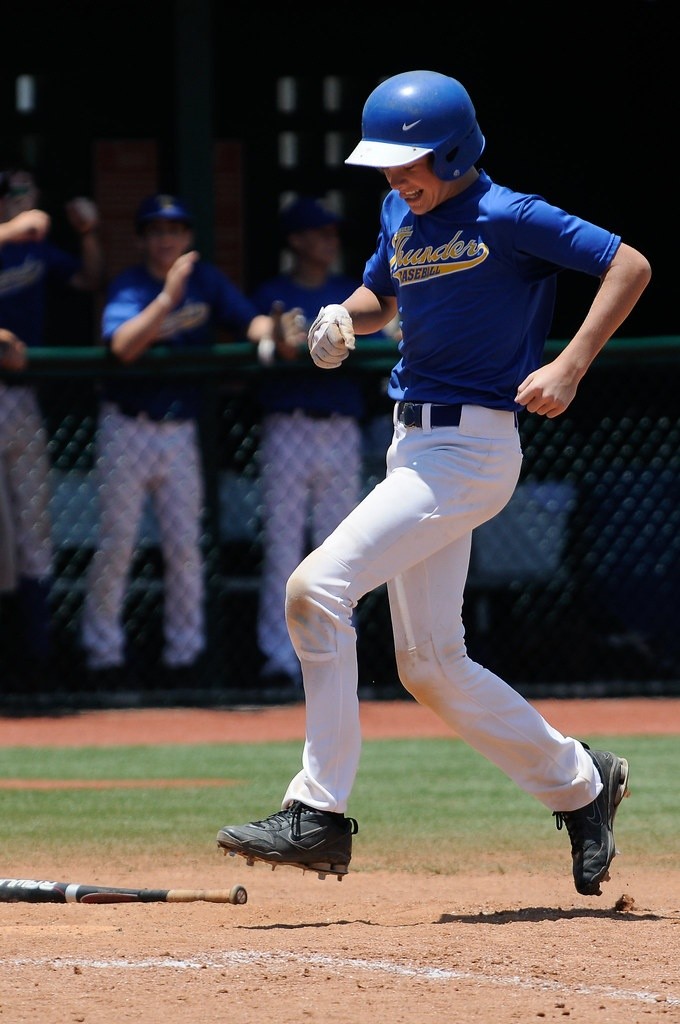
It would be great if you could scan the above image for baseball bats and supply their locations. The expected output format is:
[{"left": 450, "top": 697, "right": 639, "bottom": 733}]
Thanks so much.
[{"left": 0, "top": 878, "right": 247, "bottom": 904}]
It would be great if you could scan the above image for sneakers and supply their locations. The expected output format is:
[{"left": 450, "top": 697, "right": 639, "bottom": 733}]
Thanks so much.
[
  {"left": 552, "top": 741, "right": 631, "bottom": 897},
  {"left": 217, "top": 801, "right": 358, "bottom": 882}
]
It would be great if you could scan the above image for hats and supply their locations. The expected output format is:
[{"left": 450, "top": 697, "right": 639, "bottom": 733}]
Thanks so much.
[
  {"left": 280, "top": 198, "right": 344, "bottom": 236},
  {"left": 133, "top": 192, "right": 195, "bottom": 235},
  {"left": 345, "top": 70, "right": 486, "bottom": 181}
]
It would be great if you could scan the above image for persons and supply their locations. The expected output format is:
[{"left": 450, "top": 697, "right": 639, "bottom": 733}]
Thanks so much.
[
  {"left": 215, "top": 69, "right": 651, "bottom": 898},
  {"left": 0, "top": 108, "right": 379, "bottom": 699}
]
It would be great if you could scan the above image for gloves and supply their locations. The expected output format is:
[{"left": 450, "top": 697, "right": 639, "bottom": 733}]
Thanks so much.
[
  {"left": 258, "top": 301, "right": 307, "bottom": 364},
  {"left": 307, "top": 303, "right": 356, "bottom": 369}
]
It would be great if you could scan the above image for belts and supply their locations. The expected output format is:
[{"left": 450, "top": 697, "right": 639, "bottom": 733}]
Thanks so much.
[{"left": 398, "top": 400, "right": 518, "bottom": 429}]
